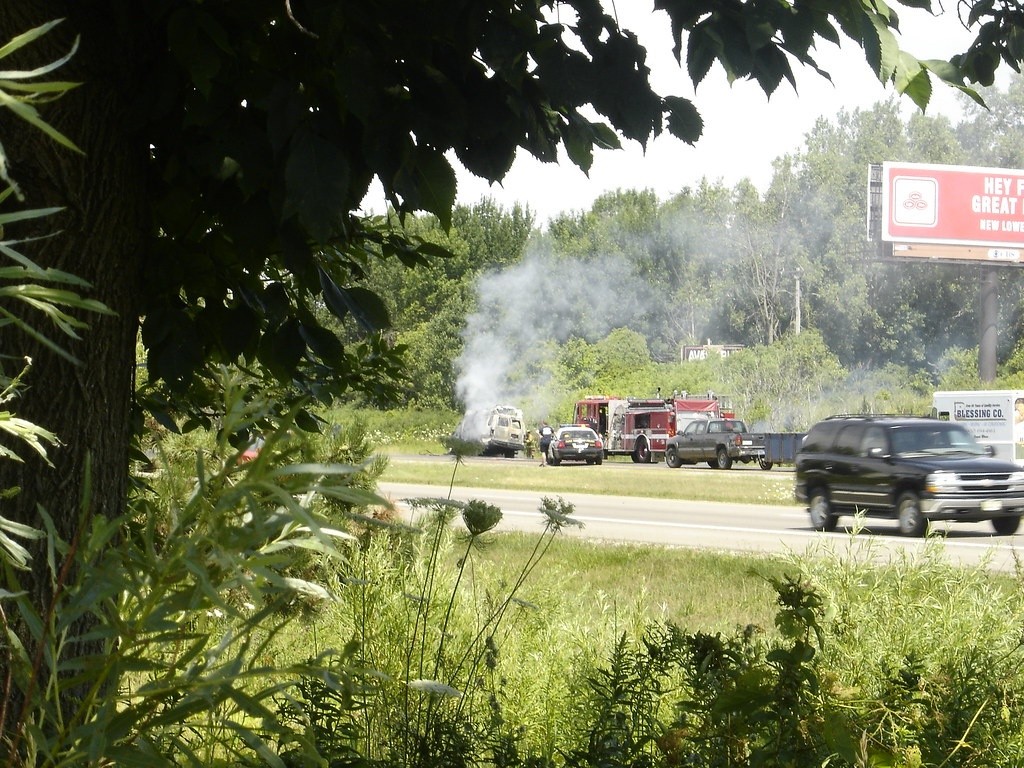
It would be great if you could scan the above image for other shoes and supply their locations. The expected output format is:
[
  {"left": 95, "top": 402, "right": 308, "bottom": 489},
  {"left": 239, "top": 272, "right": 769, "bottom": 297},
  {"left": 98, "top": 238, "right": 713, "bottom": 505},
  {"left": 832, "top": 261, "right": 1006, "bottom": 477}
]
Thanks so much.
[{"left": 539, "top": 463, "right": 546, "bottom": 467}]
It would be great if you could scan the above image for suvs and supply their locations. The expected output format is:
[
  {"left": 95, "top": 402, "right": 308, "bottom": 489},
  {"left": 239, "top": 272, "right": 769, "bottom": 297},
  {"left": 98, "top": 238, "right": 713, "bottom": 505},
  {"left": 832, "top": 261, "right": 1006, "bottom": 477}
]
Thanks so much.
[{"left": 792, "top": 413, "right": 1024, "bottom": 537}]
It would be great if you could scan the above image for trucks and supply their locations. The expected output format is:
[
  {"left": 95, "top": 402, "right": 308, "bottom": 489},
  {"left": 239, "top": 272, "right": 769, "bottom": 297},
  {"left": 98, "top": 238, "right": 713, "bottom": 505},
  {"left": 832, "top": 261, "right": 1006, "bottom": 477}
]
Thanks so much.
[{"left": 448, "top": 413, "right": 527, "bottom": 460}]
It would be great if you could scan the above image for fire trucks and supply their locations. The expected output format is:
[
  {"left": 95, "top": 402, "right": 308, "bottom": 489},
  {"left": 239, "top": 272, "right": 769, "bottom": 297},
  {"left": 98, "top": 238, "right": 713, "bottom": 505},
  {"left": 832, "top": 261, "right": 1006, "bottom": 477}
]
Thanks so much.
[{"left": 572, "top": 387, "right": 734, "bottom": 464}]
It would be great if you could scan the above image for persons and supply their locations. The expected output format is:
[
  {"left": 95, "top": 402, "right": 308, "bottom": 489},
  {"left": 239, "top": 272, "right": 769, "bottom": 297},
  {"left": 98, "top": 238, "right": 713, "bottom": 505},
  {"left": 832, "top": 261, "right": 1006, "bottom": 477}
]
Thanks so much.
[
  {"left": 539, "top": 421, "right": 555, "bottom": 467},
  {"left": 523, "top": 430, "right": 534, "bottom": 458}
]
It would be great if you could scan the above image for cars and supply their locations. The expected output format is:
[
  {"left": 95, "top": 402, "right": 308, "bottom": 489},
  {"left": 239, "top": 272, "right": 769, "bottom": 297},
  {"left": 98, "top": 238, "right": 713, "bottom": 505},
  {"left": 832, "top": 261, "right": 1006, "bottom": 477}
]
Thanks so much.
[{"left": 547, "top": 424, "right": 603, "bottom": 466}]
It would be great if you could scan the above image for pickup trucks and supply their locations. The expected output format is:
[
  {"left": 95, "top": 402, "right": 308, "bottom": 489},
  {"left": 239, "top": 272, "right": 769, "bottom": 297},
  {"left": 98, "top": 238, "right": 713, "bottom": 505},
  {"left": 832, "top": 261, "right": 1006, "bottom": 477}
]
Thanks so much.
[{"left": 665, "top": 418, "right": 772, "bottom": 469}]
